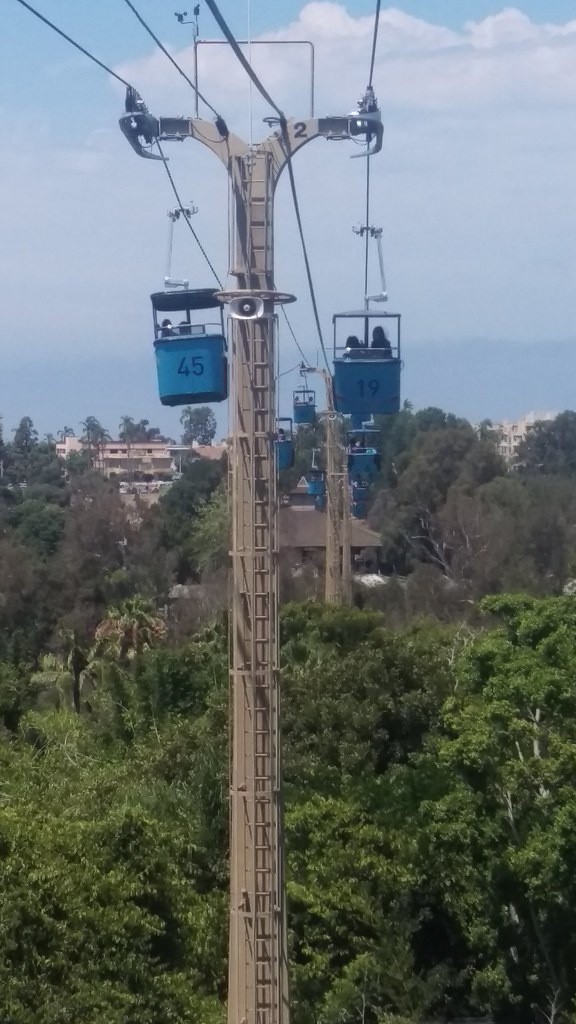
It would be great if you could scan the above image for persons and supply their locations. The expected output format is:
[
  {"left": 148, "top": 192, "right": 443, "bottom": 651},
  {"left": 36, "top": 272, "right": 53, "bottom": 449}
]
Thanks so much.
[
  {"left": 279, "top": 429, "right": 285, "bottom": 442},
  {"left": 343, "top": 336, "right": 362, "bottom": 358},
  {"left": 295, "top": 396, "right": 300, "bottom": 405},
  {"left": 371, "top": 327, "right": 392, "bottom": 358},
  {"left": 350, "top": 440, "right": 360, "bottom": 453},
  {"left": 161, "top": 319, "right": 178, "bottom": 337},
  {"left": 306, "top": 396, "right": 314, "bottom": 405}
]
